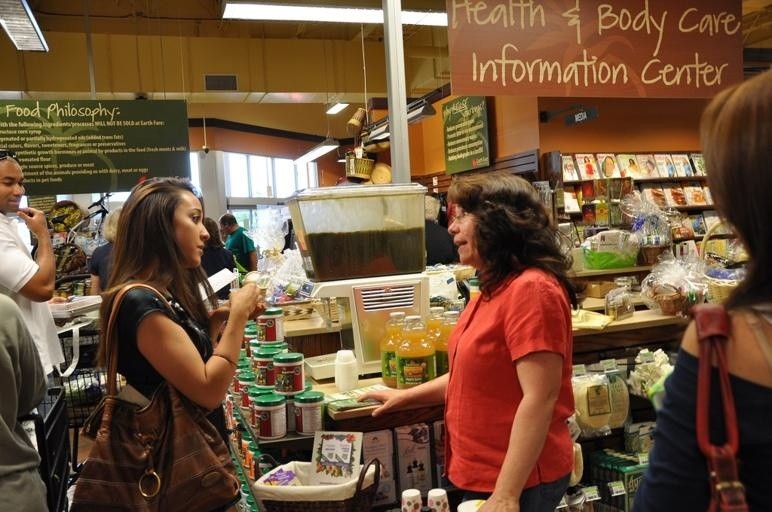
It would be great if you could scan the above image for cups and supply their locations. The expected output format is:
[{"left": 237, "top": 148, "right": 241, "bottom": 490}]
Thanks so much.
[{"left": 399, "top": 488, "right": 451, "bottom": 512}]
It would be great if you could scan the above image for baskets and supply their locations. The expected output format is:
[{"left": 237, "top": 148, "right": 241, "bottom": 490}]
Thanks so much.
[
  {"left": 265, "top": 301, "right": 313, "bottom": 321},
  {"left": 700, "top": 217, "right": 743, "bottom": 304},
  {"left": 311, "top": 299, "right": 338, "bottom": 322},
  {"left": 347, "top": 107, "right": 366, "bottom": 135},
  {"left": 346, "top": 156, "right": 375, "bottom": 180},
  {"left": 637, "top": 244, "right": 673, "bottom": 266},
  {"left": 251, "top": 454, "right": 383, "bottom": 512},
  {"left": 654, "top": 284, "right": 702, "bottom": 316}
]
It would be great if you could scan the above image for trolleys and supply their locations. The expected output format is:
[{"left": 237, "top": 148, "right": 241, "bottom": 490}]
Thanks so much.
[
  {"left": 51, "top": 319, "right": 123, "bottom": 490},
  {"left": 17, "top": 385, "right": 72, "bottom": 512},
  {"left": 216, "top": 389, "right": 383, "bottom": 511}
]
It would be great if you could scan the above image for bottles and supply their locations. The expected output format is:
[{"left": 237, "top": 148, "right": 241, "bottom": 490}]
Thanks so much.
[
  {"left": 379, "top": 306, "right": 460, "bottom": 391},
  {"left": 333, "top": 349, "right": 360, "bottom": 395}
]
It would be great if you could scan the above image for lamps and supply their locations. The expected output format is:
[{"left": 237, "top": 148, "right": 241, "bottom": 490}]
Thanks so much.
[
  {"left": 364, "top": 91, "right": 436, "bottom": 140},
  {"left": 289, "top": 37, "right": 342, "bottom": 174},
  {"left": 215, "top": 1, "right": 452, "bottom": 32},
  {"left": 322, "top": 41, "right": 350, "bottom": 114},
  {"left": 0, "top": 0, "right": 49, "bottom": 53}
]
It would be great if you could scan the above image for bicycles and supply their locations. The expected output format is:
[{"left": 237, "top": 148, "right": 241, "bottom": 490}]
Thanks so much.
[{"left": 30, "top": 192, "right": 112, "bottom": 263}]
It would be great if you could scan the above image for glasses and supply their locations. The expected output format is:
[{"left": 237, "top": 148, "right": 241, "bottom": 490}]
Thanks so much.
[{"left": 0, "top": 151, "right": 19, "bottom": 162}]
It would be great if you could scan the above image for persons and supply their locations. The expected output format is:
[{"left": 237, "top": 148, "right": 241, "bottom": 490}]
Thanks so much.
[
  {"left": 350, "top": 169, "right": 589, "bottom": 512},
  {"left": 626, "top": 63, "right": 770, "bottom": 512},
  {"left": 1, "top": 146, "right": 272, "bottom": 512}
]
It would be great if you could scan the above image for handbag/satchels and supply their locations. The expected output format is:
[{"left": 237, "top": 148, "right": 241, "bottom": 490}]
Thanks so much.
[{"left": 66, "top": 379, "right": 240, "bottom": 512}]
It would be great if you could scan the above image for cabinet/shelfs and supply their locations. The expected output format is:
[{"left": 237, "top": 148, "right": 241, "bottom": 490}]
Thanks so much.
[{"left": 541, "top": 150, "right": 750, "bottom": 279}]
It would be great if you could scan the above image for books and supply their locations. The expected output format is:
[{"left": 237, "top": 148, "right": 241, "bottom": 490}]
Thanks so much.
[{"left": 561, "top": 150, "right": 749, "bottom": 273}]
